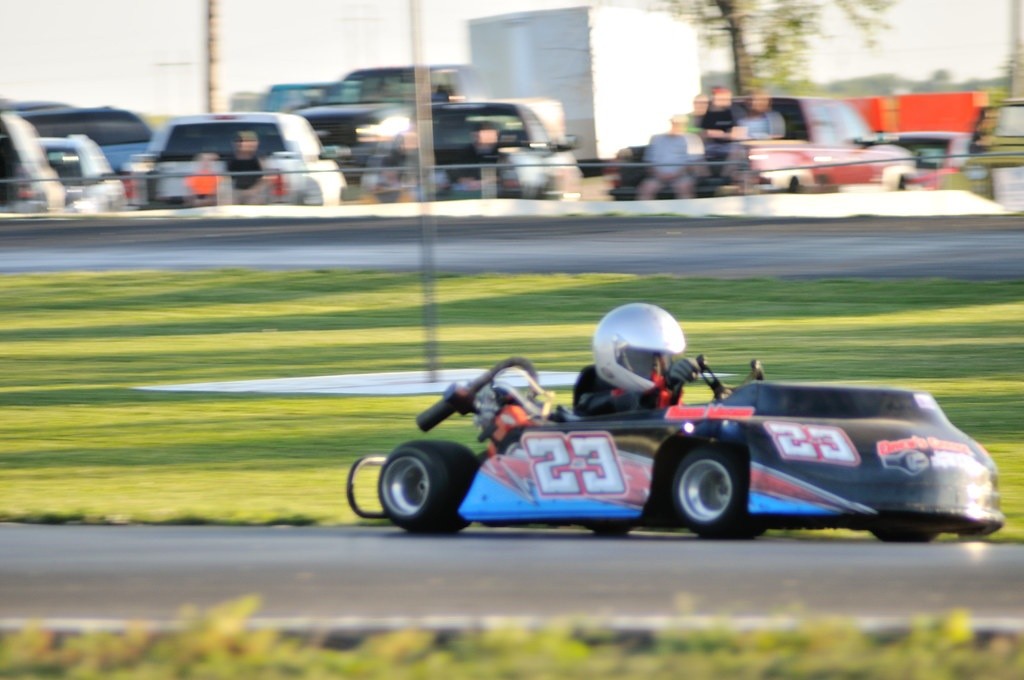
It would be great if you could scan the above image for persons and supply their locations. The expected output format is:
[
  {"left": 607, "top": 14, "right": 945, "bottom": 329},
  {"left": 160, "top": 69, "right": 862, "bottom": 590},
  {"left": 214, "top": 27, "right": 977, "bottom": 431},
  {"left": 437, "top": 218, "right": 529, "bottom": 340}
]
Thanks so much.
[
  {"left": 635, "top": 84, "right": 788, "bottom": 201},
  {"left": 222, "top": 128, "right": 274, "bottom": 205},
  {"left": 572, "top": 300, "right": 705, "bottom": 419},
  {"left": 379, "top": 128, "right": 508, "bottom": 201}
]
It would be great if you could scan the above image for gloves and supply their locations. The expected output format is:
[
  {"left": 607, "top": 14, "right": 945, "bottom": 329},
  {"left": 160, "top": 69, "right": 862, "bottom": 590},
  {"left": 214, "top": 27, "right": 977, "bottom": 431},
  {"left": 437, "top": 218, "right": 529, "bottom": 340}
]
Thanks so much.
[{"left": 665, "top": 359, "right": 699, "bottom": 386}]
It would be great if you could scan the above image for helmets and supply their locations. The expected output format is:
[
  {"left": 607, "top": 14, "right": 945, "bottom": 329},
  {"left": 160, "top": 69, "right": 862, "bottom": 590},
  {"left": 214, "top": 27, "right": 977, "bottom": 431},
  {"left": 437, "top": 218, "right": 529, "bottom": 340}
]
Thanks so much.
[{"left": 592, "top": 302, "right": 686, "bottom": 391}]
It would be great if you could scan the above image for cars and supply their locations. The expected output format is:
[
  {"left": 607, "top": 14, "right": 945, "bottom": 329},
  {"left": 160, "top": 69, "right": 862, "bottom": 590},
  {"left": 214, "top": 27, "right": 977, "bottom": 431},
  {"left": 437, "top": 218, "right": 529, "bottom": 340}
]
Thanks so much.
[{"left": 1, "top": 63, "right": 928, "bottom": 215}]
